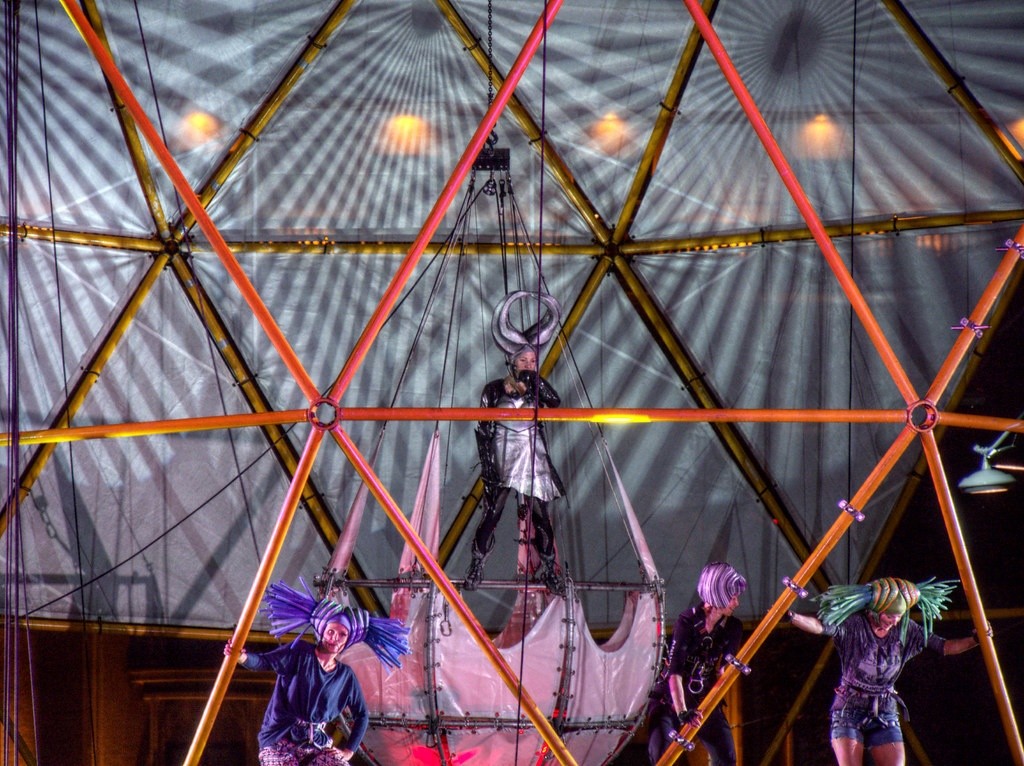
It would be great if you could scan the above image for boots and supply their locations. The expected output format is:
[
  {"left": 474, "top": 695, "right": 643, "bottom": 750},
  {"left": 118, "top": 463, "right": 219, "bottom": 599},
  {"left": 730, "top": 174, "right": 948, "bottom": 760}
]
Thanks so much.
[
  {"left": 540, "top": 552, "right": 564, "bottom": 595},
  {"left": 464, "top": 534, "right": 494, "bottom": 590}
]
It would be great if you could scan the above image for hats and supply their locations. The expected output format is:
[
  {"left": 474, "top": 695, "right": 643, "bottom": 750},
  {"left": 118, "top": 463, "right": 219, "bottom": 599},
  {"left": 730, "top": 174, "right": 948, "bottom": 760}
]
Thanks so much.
[
  {"left": 811, "top": 577, "right": 961, "bottom": 648},
  {"left": 491, "top": 291, "right": 560, "bottom": 360},
  {"left": 697, "top": 563, "right": 747, "bottom": 610},
  {"left": 258, "top": 575, "right": 412, "bottom": 674}
]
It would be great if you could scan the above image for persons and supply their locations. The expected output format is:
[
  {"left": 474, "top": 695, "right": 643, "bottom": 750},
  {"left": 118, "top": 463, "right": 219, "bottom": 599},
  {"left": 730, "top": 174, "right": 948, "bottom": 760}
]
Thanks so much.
[
  {"left": 463, "top": 345, "right": 568, "bottom": 596},
  {"left": 648, "top": 561, "right": 747, "bottom": 766},
  {"left": 767, "top": 576, "right": 994, "bottom": 766},
  {"left": 223, "top": 576, "right": 413, "bottom": 766}
]
